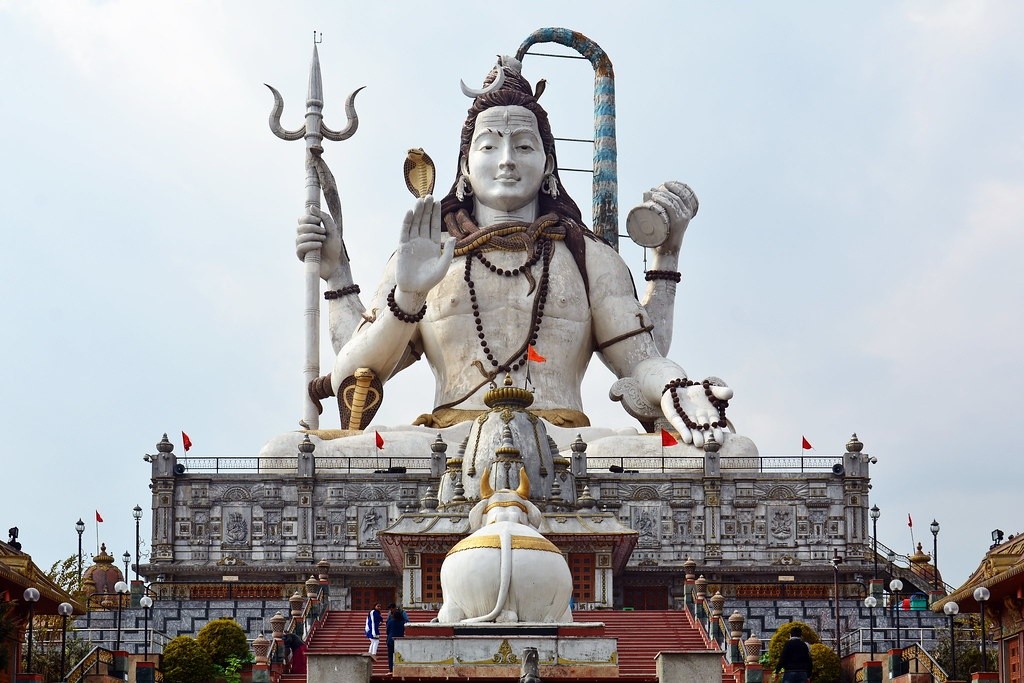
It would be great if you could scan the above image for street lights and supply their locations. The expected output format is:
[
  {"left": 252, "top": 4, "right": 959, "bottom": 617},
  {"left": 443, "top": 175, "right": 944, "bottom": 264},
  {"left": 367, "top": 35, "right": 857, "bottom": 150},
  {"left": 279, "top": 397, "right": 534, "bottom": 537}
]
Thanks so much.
[
  {"left": 130, "top": 504, "right": 145, "bottom": 609},
  {"left": 869, "top": 504, "right": 884, "bottom": 607},
  {"left": 57, "top": 602, "right": 74, "bottom": 683},
  {"left": 75, "top": 518, "right": 86, "bottom": 591},
  {"left": 23, "top": 587, "right": 41, "bottom": 674},
  {"left": 108, "top": 580, "right": 130, "bottom": 673},
  {"left": 863, "top": 596, "right": 884, "bottom": 683},
  {"left": 970, "top": 586, "right": 999, "bottom": 683},
  {"left": 943, "top": 602, "right": 967, "bottom": 683},
  {"left": 889, "top": 578, "right": 903, "bottom": 679},
  {"left": 928, "top": 519, "right": 944, "bottom": 606},
  {"left": 122, "top": 550, "right": 131, "bottom": 584},
  {"left": 136, "top": 595, "right": 155, "bottom": 683}
]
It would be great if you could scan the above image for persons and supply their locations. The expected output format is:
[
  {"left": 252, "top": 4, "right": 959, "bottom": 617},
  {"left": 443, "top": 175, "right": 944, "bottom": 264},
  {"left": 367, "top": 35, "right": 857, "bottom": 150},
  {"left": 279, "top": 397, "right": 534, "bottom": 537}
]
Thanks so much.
[
  {"left": 365, "top": 601, "right": 384, "bottom": 656},
  {"left": 256, "top": 62, "right": 761, "bottom": 468},
  {"left": 774, "top": 627, "right": 813, "bottom": 683},
  {"left": 384, "top": 603, "right": 409, "bottom": 674}
]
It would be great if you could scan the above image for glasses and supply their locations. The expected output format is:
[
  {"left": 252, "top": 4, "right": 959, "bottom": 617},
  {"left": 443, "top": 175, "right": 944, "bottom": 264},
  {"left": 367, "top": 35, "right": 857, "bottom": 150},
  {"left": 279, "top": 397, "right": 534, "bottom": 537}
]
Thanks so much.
[{"left": 388, "top": 607, "right": 394, "bottom": 609}]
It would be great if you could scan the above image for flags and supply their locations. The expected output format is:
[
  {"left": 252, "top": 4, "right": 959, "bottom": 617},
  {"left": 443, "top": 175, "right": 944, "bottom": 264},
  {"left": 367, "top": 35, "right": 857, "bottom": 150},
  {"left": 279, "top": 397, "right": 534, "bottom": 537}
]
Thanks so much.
[
  {"left": 376, "top": 431, "right": 383, "bottom": 449},
  {"left": 96, "top": 512, "right": 103, "bottom": 523},
  {"left": 183, "top": 432, "right": 191, "bottom": 451},
  {"left": 803, "top": 438, "right": 812, "bottom": 450}
]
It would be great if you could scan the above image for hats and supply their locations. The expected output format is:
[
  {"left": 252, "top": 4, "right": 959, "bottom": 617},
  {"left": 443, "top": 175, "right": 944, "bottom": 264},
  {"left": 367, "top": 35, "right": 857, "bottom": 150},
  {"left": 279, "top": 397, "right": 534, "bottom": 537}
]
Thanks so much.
[{"left": 386, "top": 602, "right": 396, "bottom": 607}]
